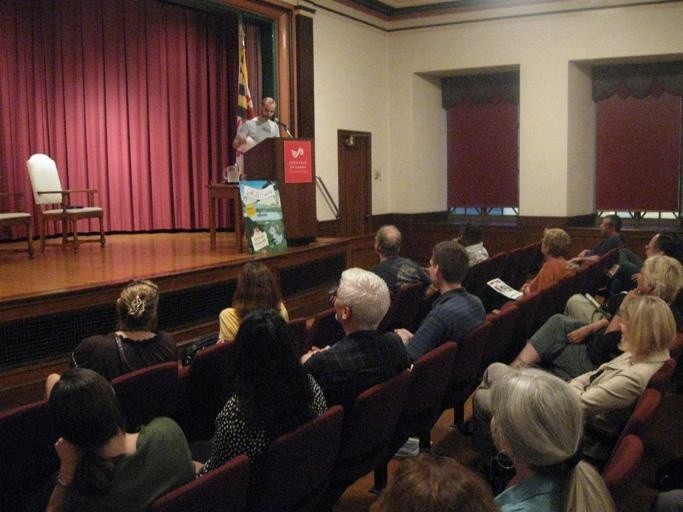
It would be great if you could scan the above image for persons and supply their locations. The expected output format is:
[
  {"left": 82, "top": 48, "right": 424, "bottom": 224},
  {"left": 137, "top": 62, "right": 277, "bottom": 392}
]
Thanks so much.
[
  {"left": 45, "top": 277, "right": 176, "bottom": 397},
  {"left": 218, "top": 262, "right": 289, "bottom": 344},
  {"left": 189, "top": 306, "right": 327, "bottom": 478},
  {"left": 302, "top": 214, "right": 681, "bottom": 511},
  {"left": 232, "top": 94, "right": 281, "bottom": 174},
  {"left": 44, "top": 368, "right": 196, "bottom": 512}
]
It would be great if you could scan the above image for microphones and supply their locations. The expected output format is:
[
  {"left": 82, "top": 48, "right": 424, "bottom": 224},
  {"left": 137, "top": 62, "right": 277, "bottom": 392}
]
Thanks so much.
[{"left": 269, "top": 114, "right": 280, "bottom": 123}]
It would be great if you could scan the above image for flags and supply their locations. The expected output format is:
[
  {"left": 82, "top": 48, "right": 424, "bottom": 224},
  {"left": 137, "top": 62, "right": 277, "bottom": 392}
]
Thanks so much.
[{"left": 234, "top": 31, "right": 255, "bottom": 131}]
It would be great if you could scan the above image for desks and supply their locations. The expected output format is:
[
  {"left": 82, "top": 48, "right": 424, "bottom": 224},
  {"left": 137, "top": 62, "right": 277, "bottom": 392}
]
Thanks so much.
[{"left": 204, "top": 183, "right": 245, "bottom": 253}]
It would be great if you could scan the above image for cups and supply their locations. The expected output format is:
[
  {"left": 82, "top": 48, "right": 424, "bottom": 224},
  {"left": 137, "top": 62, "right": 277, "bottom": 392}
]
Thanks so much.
[{"left": 222, "top": 165, "right": 239, "bottom": 184}]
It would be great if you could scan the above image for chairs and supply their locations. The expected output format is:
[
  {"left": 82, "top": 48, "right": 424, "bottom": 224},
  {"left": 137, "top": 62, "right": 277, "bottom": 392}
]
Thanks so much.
[
  {"left": 0, "top": 191, "right": 35, "bottom": 259},
  {"left": 0, "top": 240, "right": 683, "bottom": 511},
  {"left": 25, "top": 153, "right": 105, "bottom": 253}
]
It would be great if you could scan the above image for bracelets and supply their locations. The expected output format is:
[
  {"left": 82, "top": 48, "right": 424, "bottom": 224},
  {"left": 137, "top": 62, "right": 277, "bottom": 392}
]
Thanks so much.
[{"left": 55, "top": 474, "right": 67, "bottom": 489}]
